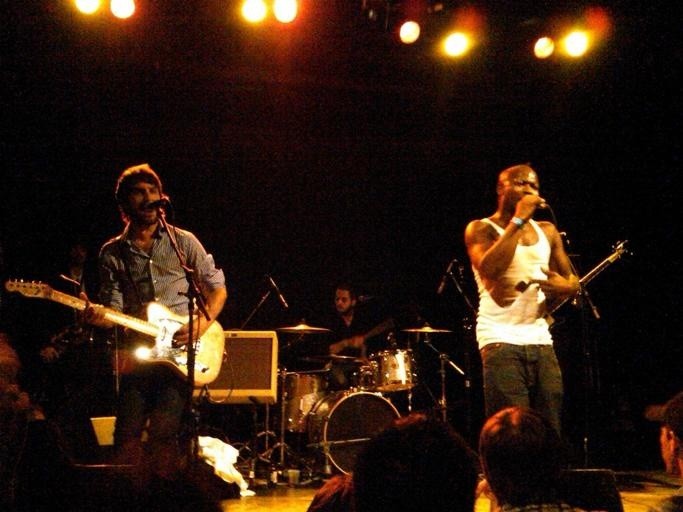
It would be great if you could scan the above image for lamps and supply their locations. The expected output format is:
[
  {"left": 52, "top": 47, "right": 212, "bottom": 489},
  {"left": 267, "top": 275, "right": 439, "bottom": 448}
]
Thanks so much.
[{"left": 369, "top": 1, "right": 423, "bottom": 45}]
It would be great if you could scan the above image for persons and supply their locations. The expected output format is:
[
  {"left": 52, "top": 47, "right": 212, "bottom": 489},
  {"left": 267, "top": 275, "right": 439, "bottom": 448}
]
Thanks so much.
[
  {"left": 47, "top": 235, "right": 102, "bottom": 335},
  {"left": 2, "top": 330, "right": 240, "bottom": 512},
  {"left": 322, "top": 282, "right": 373, "bottom": 354},
  {"left": 309, "top": 405, "right": 609, "bottom": 512},
  {"left": 646, "top": 394, "right": 682, "bottom": 512},
  {"left": 79, "top": 163, "right": 228, "bottom": 465},
  {"left": 464, "top": 164, "right": 580, "bottom": 454}
]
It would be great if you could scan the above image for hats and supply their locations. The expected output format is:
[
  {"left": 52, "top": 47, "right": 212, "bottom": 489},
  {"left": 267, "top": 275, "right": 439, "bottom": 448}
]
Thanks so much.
[{"left": 646, "top": 394, "right": 682, "bottom": 427}]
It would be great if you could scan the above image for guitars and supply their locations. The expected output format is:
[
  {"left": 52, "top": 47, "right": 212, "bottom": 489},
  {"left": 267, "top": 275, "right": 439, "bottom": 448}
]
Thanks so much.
[
  {"left": 3, "top": 278, "right": 224, "bottom": 386},
  {"left": 540, "top": 239, "right": 632, "bottom": 326}
]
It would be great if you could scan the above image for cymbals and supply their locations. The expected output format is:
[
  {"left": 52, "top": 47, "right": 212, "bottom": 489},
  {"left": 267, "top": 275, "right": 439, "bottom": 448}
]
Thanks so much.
[
  {"left": 401, "top": 326, "right": 451, "bottom": 332},
  {"left": 277, "top": 324, "right": 329, "bottom": 334}
]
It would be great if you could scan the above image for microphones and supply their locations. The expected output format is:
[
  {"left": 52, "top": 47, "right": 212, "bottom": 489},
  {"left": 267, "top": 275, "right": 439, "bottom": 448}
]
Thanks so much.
[
  {"left": 358, "top": 292, "right": 385, "bottom": 304},
  {"left": 435, "top": 258, "right": 458, "bottom": 294},
  {"left": 536, "top": 201, "right": 548, "bottom": 209},
  {"left": 264, "top": 272, "right": 290, "bottom": 311},
  {"left": 143, "top": 196, "right": 168, "bottom": 213}
]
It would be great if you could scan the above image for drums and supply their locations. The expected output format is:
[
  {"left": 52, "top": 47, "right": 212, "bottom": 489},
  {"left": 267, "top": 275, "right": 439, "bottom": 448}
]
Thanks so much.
[
  {"left": 306, "top": 390, "right": 400, "bottom": 473},
  {"left": 368, "top": 348, "right": 419, "bottom": 392},
  {"left": 284, "top": 374, "right": 329, "bottom": 433}
]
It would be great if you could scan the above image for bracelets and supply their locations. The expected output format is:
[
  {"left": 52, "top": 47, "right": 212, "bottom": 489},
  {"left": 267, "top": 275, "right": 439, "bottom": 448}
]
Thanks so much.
[{"left": 508, "top": 216, "right": 524, "bottom": 228}]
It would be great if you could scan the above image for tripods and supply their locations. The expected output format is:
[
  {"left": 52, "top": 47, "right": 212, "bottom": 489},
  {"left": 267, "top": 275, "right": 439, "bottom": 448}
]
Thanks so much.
[{"left": 251, "top": 373, "right": 313, "bottom": 468}]
